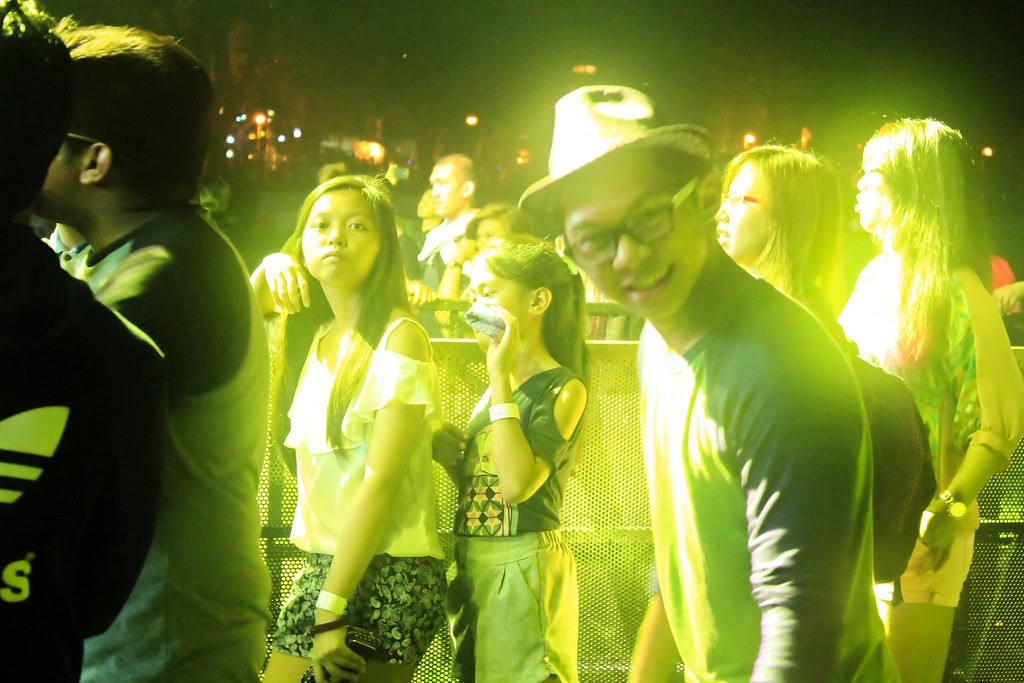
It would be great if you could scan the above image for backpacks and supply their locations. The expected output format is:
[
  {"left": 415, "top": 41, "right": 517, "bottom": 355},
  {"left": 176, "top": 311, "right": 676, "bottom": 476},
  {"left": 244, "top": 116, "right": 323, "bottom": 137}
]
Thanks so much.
[{"left": 850, "top": 355, "right": 937, "bottom": 585}]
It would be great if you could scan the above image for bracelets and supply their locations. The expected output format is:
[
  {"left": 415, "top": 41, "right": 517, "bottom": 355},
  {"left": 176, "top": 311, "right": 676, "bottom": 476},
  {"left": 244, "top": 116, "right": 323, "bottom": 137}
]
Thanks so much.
[
  {"left": 309, "top": 618, "right": 350, "bottom": 634},
  {"left": 315, "top": 591, "right": 349, "bottom": 616},
  {"left": 489, "top": 403, "right": 521, "bottom": 422}
]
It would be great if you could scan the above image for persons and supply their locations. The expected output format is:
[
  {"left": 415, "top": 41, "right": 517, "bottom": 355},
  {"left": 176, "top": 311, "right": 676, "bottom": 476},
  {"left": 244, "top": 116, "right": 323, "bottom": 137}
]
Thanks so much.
[
  {"left": 518, "top": 84, "right": 898, "bottom": 683},
  {"left": 200, "top": 149, "right": 644, "bottom": 682},
  {"left": 31, "top": 22, "right": 270, "bottom": 683},
  {"left": 701, "top": 120, "right": 1024, "bottom": 683},
  {"left": 0, "top": 0, "right": 167, "bottom": 683}
]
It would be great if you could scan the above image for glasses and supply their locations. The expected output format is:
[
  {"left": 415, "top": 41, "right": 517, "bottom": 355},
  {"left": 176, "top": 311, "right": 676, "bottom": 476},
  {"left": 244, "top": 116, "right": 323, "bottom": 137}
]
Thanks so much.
[
  {"left": 858, "top": 168, "right": 885, "bottom": 181},
  {"left": 721, "top": 192, "right": 764, "bottom": 208},
  {"left": 563, "top": 173, "right": 700, "bottom": 270}
]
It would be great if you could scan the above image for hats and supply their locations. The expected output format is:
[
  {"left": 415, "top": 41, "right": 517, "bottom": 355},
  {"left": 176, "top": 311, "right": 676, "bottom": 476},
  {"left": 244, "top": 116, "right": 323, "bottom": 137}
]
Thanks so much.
[{"left": 518, "top": 85, "right": 715, "bottom": 230}]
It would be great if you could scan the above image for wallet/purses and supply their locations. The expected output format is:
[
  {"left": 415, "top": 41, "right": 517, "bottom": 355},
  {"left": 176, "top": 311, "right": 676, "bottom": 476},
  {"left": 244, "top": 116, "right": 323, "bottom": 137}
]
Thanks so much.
[{"left": 299, "top": 626, "right": 378, "bottom": 683}]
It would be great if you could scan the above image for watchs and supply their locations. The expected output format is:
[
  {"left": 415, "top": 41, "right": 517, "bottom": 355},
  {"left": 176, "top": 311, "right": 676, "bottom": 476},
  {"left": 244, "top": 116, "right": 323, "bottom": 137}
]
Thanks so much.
[{"left": 939, "top": 491, "right": 968, "bottom": 521}]
